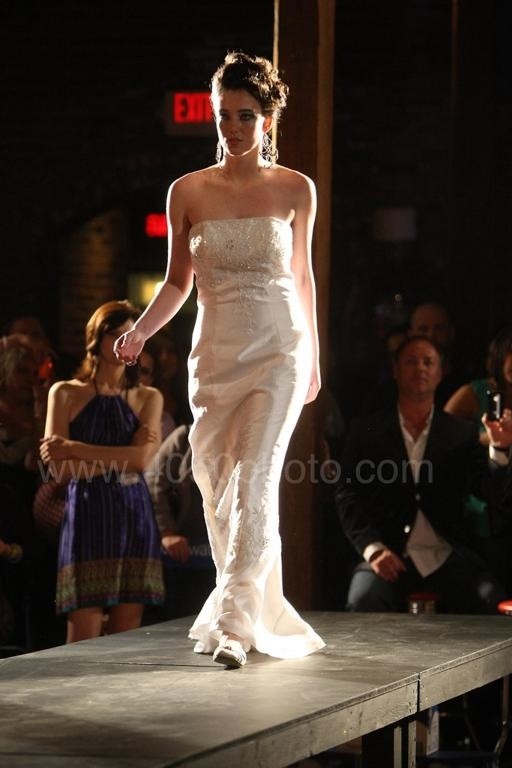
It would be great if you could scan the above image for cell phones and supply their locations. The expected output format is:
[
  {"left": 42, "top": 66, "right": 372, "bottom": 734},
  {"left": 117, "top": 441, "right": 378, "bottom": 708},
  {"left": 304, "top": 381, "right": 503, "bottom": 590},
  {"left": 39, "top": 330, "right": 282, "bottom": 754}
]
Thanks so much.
[{"left": 486, "top": 389, "right": 503, "bottom": 421}]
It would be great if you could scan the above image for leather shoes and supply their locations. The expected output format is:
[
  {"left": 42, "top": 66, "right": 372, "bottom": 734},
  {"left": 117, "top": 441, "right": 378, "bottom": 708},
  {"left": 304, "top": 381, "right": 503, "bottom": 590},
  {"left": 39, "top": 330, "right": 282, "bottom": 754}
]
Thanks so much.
[{"left": 213, "top": 640, "right": 247, "bottom": 668}]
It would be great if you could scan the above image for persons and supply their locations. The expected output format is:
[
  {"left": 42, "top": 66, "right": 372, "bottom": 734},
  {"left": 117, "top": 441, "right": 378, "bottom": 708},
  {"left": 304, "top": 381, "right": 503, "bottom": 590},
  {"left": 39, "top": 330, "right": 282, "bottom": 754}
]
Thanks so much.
[
  {"left": 110, "top": 47, "right": 329, "bottom": 673},
  {"left": 2, "top": 298, "right": 508, "bottom": 645}
]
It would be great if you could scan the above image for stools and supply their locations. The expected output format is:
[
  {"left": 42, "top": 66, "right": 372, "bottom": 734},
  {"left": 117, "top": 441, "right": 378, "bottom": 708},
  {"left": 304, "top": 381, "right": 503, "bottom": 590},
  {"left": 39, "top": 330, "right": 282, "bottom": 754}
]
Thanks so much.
[
  {"left": 406, "top": 589, "right": 436, "bottom": 614},
  {"left": 498, "top": 599, "right": 512, "bottom": 616}
]
